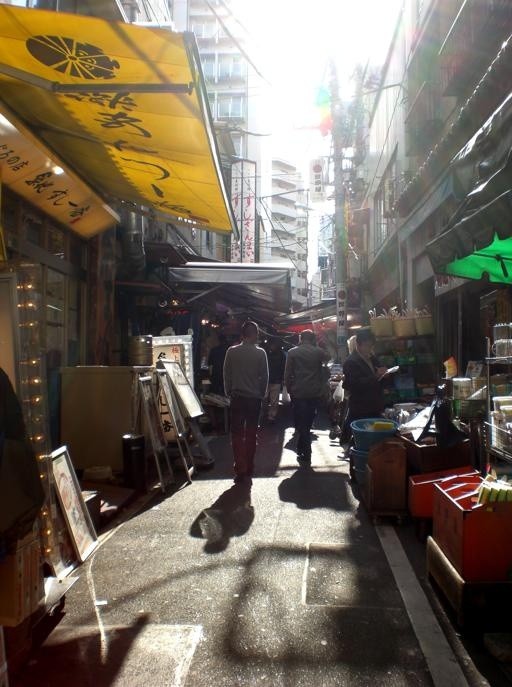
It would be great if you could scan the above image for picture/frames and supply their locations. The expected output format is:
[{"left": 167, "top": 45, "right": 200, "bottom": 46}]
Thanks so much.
[{"left": 41, "top": 359, "right": 207, "bottom": 580}]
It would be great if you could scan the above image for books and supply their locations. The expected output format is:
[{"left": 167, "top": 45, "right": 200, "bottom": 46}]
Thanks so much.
[{"left": 375, "top": 362, "right": 401, "bottom": 386}]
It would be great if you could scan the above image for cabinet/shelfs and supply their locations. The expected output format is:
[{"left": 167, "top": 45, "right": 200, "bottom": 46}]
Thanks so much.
[
  {"left": 425, "top": 535, "right": 512, "bottom": 625},
  {"left": 485, "top": 335, "right": 512, "bottom": 474},
  {"left": 366, "top": 438, "right": 407, "bottom": 526},
  {"left": 375, "top": 332, "right": 441, "bottom": 409}
]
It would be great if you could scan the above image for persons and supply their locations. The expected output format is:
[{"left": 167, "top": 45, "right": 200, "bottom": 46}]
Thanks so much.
[
  {"left": 0, "top": 366, "right": 46, "bottom": 565},
  {"left": 206, "top": 318, "right": 333, "bottom": 486},
  {"left": 342, "top": 326, "right": 389, "bottom": 459}
]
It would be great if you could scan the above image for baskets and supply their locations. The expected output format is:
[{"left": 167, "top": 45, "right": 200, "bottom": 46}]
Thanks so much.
[{"left": 368, "top": 304, "right": 435, "bottom": 337}]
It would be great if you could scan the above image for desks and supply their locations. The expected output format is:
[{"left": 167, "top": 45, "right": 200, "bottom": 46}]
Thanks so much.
[{"left": 394, "top": 432, "right": 476, "bottom": 543}]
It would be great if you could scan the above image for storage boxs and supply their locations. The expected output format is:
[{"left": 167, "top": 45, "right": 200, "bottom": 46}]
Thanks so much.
[
  {"left": 449, "top": 397, "right": 487, "bottom": 419},
  {"left": 408, "top": 466, "right": 480, "bottom": 517},
  {"left": 432, "top": 474, "right": 512, "bottom": 582}
]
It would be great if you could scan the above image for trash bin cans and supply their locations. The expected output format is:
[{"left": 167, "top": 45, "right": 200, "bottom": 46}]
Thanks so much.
[{"left": 121, "top": 432, "right": 145, "bottom": 480}]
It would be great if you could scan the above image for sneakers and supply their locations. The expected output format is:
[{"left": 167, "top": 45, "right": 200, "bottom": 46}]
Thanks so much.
[{"left": 234, "top": 455, "right": 311, "bottom": 490}]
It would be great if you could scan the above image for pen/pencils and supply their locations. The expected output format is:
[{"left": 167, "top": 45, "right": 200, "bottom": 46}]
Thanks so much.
[{"left": 375, "top": 367, "right": 388, "bottom": 372}]
